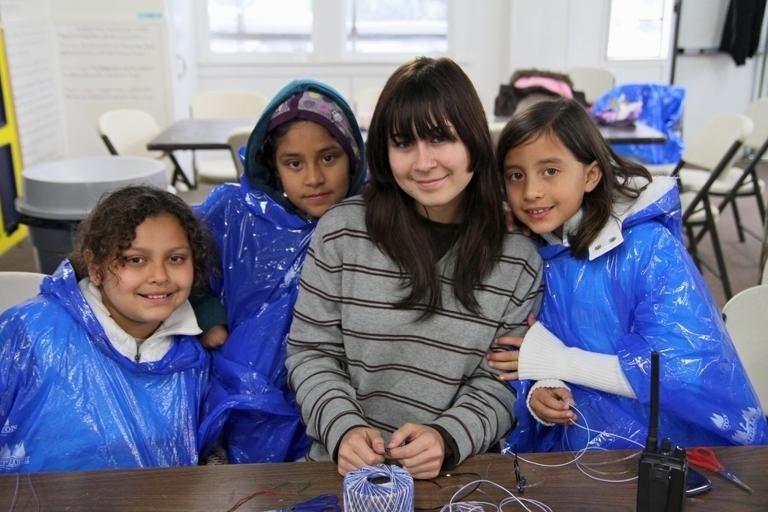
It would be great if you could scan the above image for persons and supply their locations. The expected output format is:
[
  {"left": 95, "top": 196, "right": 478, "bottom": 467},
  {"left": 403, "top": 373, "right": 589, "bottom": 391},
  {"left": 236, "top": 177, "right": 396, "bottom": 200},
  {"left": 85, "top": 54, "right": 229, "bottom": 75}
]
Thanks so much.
[
  {"left": 0, "top": 188, "right": 211, "bottom": 475},
  {"left": 190, "top": 78, "right": 364, "bottom": 463},
  {"left": 486, "top": 99, "right": 767, "bottom": 454},
  {"left": 285, "top": 56, "right": 543, "bottom": 480}
]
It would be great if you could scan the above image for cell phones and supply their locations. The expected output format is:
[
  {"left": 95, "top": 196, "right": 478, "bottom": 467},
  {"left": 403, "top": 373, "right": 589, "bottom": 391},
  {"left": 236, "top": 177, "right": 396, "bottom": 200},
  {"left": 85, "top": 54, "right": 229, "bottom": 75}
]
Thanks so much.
[{"left": 684, "top": 467, "right": 712, "bottom": 496}]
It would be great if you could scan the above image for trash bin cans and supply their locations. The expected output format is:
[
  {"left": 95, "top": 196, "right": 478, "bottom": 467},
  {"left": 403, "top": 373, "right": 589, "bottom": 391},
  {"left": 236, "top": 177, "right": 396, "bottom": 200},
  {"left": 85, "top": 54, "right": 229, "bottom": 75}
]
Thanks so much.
[{"left": 14, "top": 156, "right": 167, "bottom": 284}]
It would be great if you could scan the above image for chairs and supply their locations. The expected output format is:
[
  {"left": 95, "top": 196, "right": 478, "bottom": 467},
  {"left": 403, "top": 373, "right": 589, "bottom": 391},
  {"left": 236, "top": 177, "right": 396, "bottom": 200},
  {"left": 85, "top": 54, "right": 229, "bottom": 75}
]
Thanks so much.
[
  {"left": 718, "top": 283, "right": 767, "bottom": 415},
  {"left": 187, "top": 89, "right": 273, "bottom": 123},
  {"left": 2, "top": 270, "right": 50, "bottom": 311},
  {"left": 223, "top": 128, "right": 258, "bottom": 185},
  {"left": 574, "top": 68, "right": 614, "bottom": 107},
  {"left": 674, "top": 93, "right": 768, "bottom": 245},
  {"left": 97, "top": 109, "right": 190, "bottom": 192},
  {"left": 669, "top": 111, "right": 756, "bottom": 303}
]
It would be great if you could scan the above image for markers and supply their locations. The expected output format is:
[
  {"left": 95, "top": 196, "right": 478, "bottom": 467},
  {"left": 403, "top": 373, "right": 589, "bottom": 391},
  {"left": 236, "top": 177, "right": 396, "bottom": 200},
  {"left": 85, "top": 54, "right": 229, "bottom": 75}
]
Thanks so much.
[{"left": 677, "top": 48, "right": 711, "bottom": 54}]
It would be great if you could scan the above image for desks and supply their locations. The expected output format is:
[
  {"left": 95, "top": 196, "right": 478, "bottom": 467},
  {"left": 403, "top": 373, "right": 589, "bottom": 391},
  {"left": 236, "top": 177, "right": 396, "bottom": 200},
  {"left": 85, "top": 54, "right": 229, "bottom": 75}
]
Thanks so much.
[
  {"left": 144, "top": 116, "right": 672, "bottom": 196},
  {"left": 0, "top": 441, "right": 767, "bottom": 511}
]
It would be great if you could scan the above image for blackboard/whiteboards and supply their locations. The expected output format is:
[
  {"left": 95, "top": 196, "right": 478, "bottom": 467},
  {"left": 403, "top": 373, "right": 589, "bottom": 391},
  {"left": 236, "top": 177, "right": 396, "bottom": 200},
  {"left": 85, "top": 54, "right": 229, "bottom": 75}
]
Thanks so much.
[{"left": 674, "top": 0, "right": 767, "bottom": 55}]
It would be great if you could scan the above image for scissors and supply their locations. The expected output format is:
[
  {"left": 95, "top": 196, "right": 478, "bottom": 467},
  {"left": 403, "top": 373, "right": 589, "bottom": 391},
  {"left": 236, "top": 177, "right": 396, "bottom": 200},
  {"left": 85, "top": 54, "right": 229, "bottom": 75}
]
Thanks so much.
[
  {"left": 685, "top": 446, "right": 752, "bottom": 491},
  {"left": 263, "top": 493, "right": 342, "bottom": 512}
]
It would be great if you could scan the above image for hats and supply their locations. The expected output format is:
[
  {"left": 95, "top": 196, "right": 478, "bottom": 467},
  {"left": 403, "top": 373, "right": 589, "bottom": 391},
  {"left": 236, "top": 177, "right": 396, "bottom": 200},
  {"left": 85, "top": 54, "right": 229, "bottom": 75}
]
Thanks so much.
[{"left": 266, "top": 89, "right": 364, "bottom": 176}]
